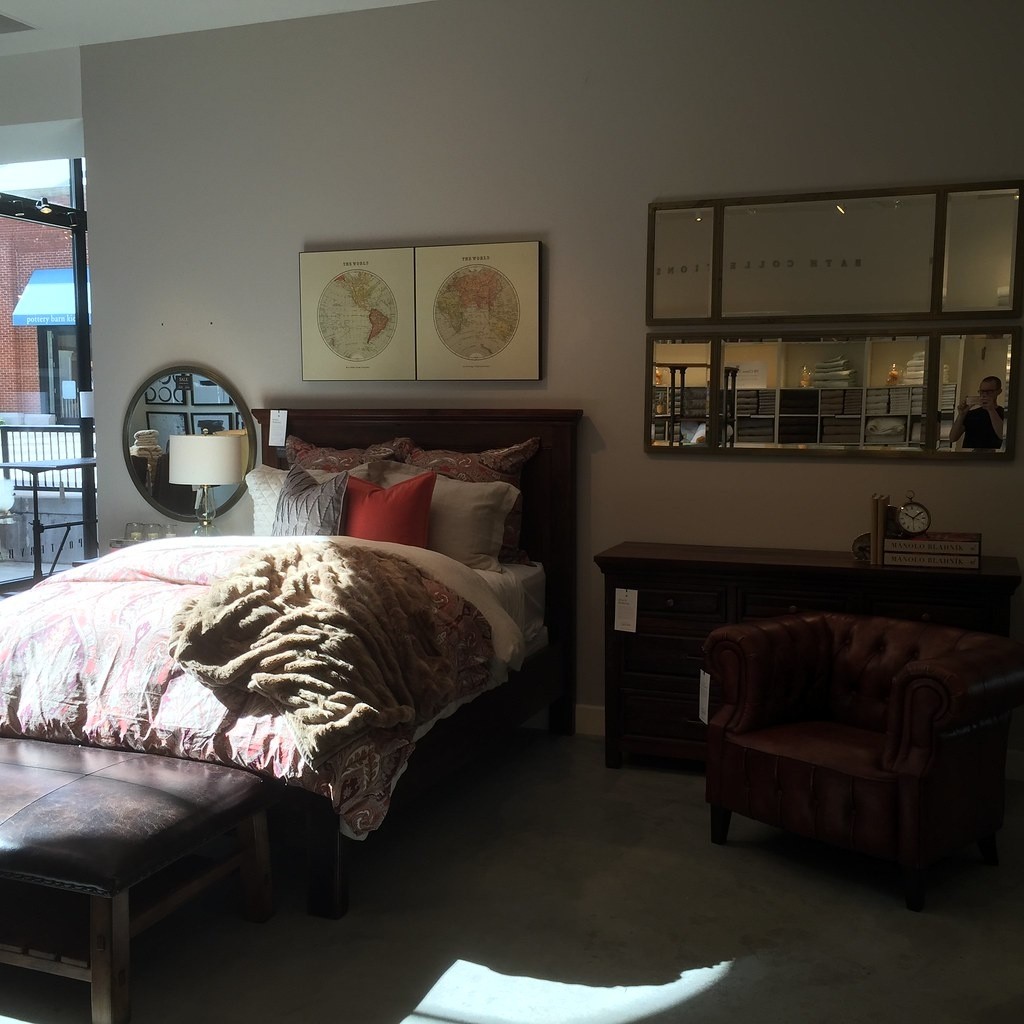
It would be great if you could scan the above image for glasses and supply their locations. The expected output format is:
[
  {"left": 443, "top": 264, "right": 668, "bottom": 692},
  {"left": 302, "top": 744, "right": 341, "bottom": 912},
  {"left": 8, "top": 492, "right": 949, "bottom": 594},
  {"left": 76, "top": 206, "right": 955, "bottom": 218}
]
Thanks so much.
[{"left": 978, "top": 389, "right": 998, "bottom": 395}]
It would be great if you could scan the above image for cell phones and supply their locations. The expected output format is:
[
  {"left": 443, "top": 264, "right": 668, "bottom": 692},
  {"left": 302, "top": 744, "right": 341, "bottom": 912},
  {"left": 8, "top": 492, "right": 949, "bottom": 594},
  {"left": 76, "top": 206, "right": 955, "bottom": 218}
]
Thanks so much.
[{"left": 966, "top": 396, "right": 983, "bottom": 405}]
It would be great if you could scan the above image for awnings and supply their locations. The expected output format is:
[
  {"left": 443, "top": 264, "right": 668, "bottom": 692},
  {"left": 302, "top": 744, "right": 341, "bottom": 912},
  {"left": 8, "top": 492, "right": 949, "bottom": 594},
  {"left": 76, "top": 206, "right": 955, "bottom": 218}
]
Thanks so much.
[{"left": 13, "top": 268, "right": 91, "bottom": 326}]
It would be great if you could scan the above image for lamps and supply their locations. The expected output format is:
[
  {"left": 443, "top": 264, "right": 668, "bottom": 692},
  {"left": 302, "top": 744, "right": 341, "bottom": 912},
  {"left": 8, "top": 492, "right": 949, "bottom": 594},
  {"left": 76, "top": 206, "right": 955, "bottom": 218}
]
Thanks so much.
[
  {"left": 212, "top": 428, "right": 249, "bottom": 479},
  {"left": 36, "top": 197, "right": 52, "bottom": 214},
  {"left": 67, "top": 212, "right": 79, "bottom": 227},
  {"left": 169, "top": 435, "right": 242, "bottom": 536},
  {"left": 13, "top": 200, "right": 25, "bottom": 216}
]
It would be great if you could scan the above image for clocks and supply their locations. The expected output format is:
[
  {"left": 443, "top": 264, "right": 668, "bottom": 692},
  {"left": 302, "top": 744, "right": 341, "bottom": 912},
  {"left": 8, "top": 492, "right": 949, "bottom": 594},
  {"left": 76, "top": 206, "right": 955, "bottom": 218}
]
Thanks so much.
[{"left": 895, "top": 489, "right": 931, "bottom": 538}]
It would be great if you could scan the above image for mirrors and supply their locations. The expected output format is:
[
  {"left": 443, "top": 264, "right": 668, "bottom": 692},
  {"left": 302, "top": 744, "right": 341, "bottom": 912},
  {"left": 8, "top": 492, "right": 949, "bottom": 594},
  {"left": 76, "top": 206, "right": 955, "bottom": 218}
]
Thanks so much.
[
  {"left": 123, "top": 365, "right": 258, "bottom": 524},
  {"left": 644, "top": 325, "right": 1022, "bottom": 463}
]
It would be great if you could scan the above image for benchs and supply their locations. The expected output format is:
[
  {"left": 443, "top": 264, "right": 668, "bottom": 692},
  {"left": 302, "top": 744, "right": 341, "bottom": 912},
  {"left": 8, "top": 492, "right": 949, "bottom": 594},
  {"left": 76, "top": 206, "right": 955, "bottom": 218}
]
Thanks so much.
[{"left": 0, "top": 737, "right": 272, "bottom": 1024}]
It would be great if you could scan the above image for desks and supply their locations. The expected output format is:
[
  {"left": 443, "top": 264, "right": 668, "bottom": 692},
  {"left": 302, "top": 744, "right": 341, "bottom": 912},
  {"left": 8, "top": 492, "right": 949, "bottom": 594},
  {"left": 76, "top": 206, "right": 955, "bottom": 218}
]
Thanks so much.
[{"left": 0, "top": 458, "right": 96, "bottom": 599}]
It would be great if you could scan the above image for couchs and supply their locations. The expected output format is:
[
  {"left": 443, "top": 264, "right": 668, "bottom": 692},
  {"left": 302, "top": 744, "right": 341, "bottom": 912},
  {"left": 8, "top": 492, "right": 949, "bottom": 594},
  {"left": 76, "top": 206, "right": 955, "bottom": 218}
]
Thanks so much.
[{"left": 700, "top": 603, "right": 1024, "bottom": 915}]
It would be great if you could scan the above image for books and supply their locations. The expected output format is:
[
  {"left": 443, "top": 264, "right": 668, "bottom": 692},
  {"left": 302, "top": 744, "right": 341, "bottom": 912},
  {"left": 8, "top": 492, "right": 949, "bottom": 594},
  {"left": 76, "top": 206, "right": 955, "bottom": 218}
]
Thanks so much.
[{"left": 884, "top": 532, "right": 981, "bottom": 569}]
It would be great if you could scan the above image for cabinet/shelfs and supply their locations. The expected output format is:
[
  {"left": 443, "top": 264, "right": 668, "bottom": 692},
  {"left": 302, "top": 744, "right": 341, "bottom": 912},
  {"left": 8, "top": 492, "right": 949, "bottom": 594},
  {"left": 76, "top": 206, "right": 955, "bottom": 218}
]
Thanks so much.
[
  {"left": 652, "top": 334, "right": 966, "bottom": 452},
  {"left": 593, "top": 542, "right": 1024, "bottom": 770}
]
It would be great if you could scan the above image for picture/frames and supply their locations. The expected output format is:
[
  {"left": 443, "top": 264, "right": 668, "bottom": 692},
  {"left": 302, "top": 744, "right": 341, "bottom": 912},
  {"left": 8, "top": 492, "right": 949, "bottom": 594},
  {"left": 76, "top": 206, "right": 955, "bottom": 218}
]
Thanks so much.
[
  {"left": 145, "top": 374, "right": 187, "bottom": 406},
  {"left": 146, "top": 410, "right": 190, "bottom": 452},
  {"left": 190, "top": 374, "right": 234, "bottom": 406},
  {"left": 191, "top": 412, "right": 233, "bottom": 435},
  {"left": 235, "top": 412, "right": 246, "bottom": 430}
]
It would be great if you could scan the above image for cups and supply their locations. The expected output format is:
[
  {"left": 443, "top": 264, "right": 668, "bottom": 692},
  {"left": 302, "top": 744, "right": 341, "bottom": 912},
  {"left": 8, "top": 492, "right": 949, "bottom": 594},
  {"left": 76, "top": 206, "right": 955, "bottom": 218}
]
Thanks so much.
[{"left": 124, "top": 522, "right": 178, "bottom": 541}]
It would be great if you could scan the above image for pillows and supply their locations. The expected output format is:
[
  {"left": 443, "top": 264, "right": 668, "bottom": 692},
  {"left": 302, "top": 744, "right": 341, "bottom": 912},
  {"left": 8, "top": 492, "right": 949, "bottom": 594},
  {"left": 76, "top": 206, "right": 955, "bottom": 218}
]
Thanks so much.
[
  {"left": 366, "top": 460, "right": 522, "bottom": 574},
  {"left": 285, "top": 435, "right": 416, "bottom": 535},
  {"left": 392, "top": 436, "right": 542, "bottom": 568},
  {"left": 245, "top": 462, "right": 371, "bottom": 536},
  {"left": 271, "top": 463, "right": 349, "bottom": 536},
  {"left": 344, "top": 471, "right": 438, "bottom": 548}
]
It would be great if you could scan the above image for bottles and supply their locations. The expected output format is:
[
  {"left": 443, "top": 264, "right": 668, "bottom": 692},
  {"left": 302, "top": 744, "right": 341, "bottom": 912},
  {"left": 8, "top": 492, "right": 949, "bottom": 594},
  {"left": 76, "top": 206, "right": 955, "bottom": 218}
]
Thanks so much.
[
  {"left": 800, "top": 365, "right": 811, "bottom": 387},
  {"left": 656, "top": 391, "right": 664, "bottom": 415},
  {"left": 655, "top": 367, "right": 663, "bottom": 385},
  {"left": 888, "top": 364, "right": 899, "bottom": 386}
]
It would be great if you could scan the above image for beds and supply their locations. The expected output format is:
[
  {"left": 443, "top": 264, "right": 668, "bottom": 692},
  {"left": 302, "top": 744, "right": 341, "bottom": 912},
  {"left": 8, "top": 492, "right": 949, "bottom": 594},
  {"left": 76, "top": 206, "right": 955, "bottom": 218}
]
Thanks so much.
[{"left": 0, "top": 408, "right": 584, "bottom": 921}]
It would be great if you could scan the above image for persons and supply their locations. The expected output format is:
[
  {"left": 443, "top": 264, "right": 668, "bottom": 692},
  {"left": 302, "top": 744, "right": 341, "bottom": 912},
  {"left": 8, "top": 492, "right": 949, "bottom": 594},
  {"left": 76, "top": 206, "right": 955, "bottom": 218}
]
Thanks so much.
[{"left": 949, "top": 376, "right": 1005, "bottom": 449}]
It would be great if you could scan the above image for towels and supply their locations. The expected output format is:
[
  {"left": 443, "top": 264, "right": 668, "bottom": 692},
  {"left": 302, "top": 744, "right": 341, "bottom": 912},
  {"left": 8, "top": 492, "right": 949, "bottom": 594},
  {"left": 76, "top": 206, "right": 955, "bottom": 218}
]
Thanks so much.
[{"left": 653, "top": 350, "right": 957, "bottom": 449}]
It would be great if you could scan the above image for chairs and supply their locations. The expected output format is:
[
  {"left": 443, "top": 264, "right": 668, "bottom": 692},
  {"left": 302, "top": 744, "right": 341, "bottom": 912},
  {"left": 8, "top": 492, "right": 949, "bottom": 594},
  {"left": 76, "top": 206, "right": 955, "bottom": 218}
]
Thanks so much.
[{"left": 155, "top": 454, "right": 195, "bottom": 514}]
